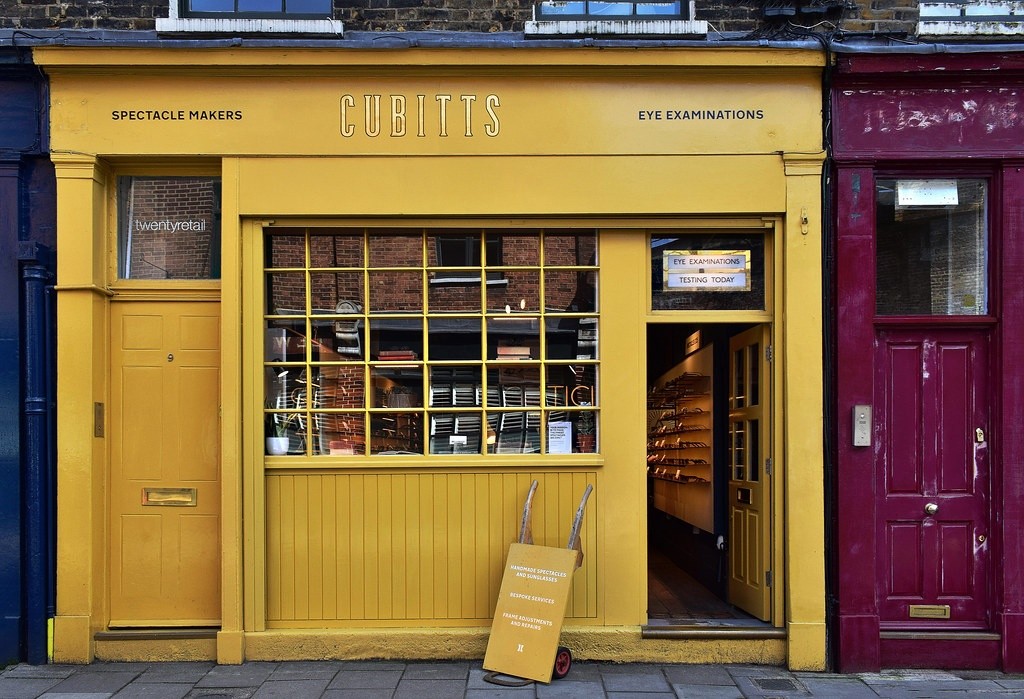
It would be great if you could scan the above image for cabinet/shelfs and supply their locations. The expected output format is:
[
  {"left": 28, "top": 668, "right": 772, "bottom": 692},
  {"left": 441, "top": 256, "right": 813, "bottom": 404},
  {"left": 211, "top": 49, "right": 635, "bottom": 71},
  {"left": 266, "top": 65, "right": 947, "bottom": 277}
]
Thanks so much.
[{"left": 647, "top": 370, "right": 711, "bottom": 487}]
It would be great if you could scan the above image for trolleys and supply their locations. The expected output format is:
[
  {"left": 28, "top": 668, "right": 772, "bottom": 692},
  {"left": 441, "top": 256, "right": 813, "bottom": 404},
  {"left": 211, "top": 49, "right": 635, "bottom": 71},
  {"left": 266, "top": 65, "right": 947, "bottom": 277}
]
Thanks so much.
[{"left": 481, "top": 480, "right": 593, "bottom": 688}]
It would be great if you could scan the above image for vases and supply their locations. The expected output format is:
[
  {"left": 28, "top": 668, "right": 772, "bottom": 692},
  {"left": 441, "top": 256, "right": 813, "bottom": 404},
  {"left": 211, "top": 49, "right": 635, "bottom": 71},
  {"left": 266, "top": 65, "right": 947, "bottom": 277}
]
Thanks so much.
[{"left": 577, "top": 433, "right": 595, "bottom": 453}]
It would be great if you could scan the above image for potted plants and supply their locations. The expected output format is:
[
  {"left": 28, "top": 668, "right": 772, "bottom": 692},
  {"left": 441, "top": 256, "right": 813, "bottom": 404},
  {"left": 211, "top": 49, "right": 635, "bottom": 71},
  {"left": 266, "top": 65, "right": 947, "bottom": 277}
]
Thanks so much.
[
  {"left": 265, "top": 396, "right": 298, "bottom": 456},
  {"left": 387, "top": 386, "right": 418, "bottom": 409}
]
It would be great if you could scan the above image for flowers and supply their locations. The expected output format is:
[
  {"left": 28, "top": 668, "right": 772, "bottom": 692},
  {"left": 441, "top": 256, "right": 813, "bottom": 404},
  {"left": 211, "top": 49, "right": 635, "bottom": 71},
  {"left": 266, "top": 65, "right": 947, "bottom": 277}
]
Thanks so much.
[{"left": 578, "top": 401, "right": 594, "bottom": 434}]
